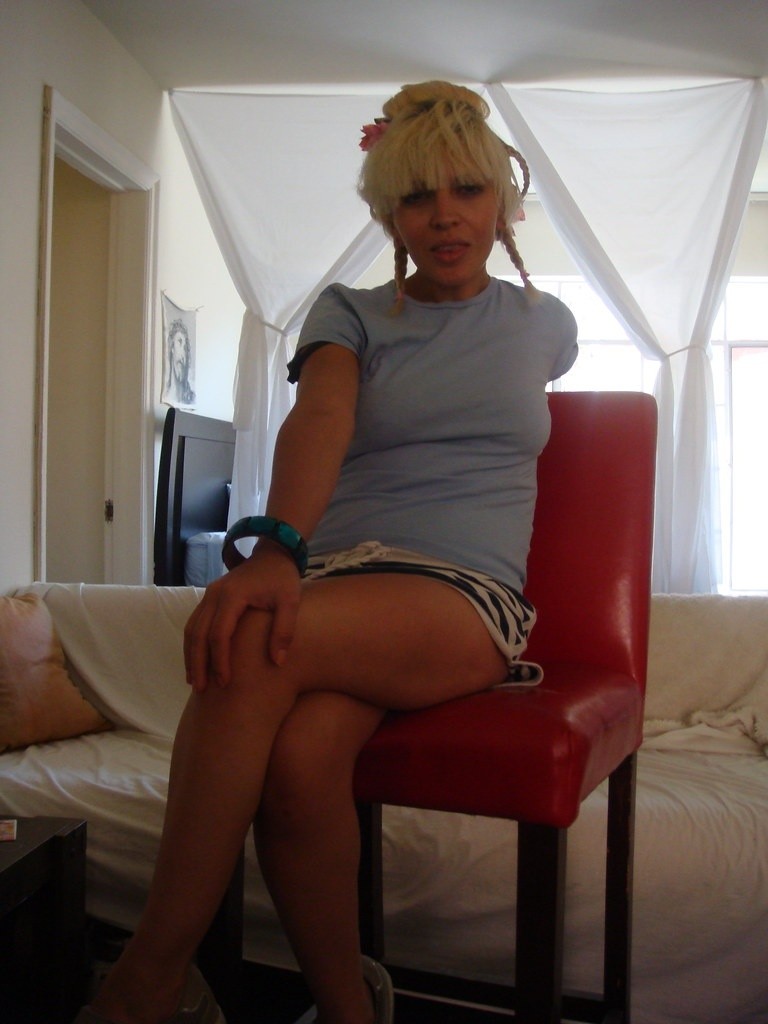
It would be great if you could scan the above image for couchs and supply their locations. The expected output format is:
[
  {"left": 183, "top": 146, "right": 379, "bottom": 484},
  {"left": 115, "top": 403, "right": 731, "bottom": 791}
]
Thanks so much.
[{"left": 0, "top": 581, "right": 768, "bottom": 1024}]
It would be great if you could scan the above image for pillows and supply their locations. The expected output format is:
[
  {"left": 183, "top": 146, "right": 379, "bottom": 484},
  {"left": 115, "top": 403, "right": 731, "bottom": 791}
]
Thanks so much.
[{"left": 0, "top": 592, "right": 116, "bottom": 753}]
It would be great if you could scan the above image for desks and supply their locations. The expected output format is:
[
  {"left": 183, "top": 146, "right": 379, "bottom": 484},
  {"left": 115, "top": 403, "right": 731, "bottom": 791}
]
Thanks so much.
[{"left": 0, "top": 815, "right": 88, "bottom": 1024}]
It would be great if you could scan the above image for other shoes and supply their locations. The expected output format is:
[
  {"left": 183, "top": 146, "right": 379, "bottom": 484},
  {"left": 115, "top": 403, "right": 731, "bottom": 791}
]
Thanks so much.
[
  {"left": 312, "top": 955, "right": 394, "bottom": 1024},
  {"left": 72, "top": 963, "right": 226, "bottom": 1024}
]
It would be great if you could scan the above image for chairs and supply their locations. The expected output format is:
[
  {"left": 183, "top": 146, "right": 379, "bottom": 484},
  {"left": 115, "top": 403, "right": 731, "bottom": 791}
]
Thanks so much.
[{"left": 195, "top": 392, "right": 658, "bottom": 1023}]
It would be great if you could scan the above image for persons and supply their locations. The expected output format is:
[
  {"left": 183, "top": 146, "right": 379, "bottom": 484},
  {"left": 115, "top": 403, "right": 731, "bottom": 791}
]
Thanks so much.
[{"left": 72, "top": 79, "right": 579, "bottom": 1024}]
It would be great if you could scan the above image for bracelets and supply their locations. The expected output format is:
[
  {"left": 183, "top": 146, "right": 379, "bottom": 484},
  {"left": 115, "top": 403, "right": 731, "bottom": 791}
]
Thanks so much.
[{"left": 221, "top": 512, "right": 312, "bottom": 575}]
